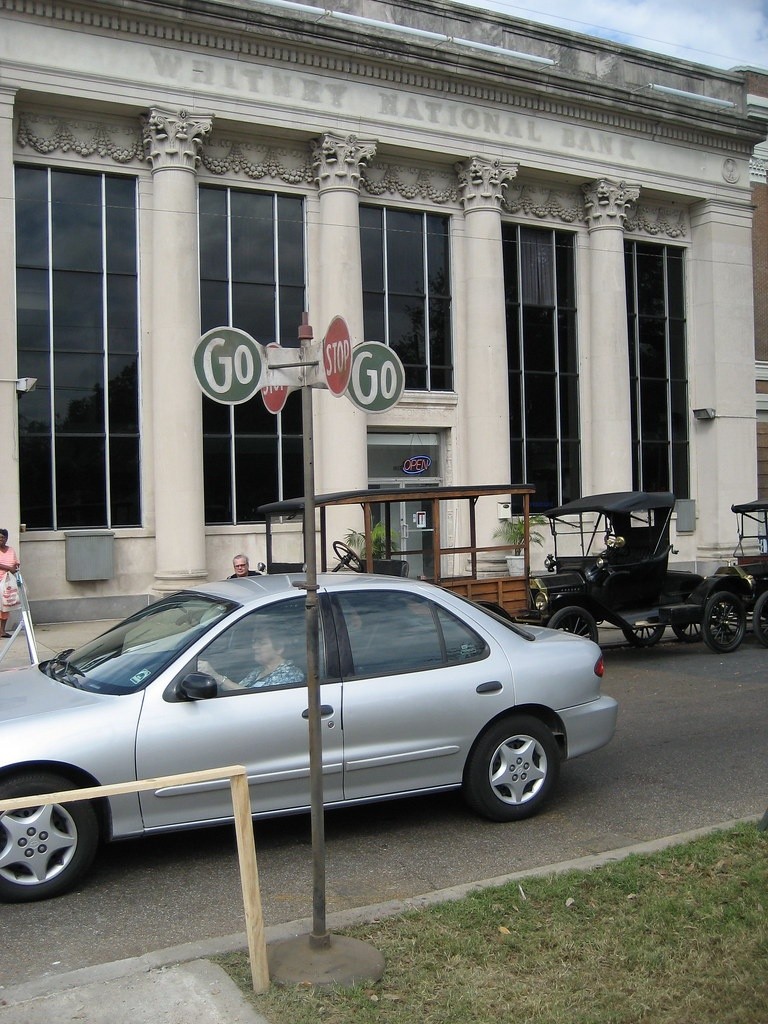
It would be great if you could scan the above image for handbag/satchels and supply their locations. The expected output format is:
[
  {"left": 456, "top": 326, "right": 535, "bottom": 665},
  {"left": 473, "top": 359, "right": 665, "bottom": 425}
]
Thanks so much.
[{"left": 0, "top": 571, "right": 22, "bottom": 613}]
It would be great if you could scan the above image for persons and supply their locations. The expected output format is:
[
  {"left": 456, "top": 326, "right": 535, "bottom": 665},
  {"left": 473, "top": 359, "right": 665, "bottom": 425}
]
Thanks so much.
[
  {"left": 0, "top": 529, "right": 20, "bottom": 639},
  {"left": 227, "top": 555, "right": 262, "bottom": 579},
  {"left": 197, "top": 618, "right": 304, "bottom": 690}
]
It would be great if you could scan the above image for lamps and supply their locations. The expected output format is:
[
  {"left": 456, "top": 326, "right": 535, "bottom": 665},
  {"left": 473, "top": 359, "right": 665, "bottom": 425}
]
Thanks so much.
[{"left": 693, "top": 408, "right": 715, "bottom": 420}]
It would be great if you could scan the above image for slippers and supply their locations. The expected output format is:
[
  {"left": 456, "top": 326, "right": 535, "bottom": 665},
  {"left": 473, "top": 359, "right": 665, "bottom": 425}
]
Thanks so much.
[{"left": 1, "top": 633, "right": 12, "bottom": 638}]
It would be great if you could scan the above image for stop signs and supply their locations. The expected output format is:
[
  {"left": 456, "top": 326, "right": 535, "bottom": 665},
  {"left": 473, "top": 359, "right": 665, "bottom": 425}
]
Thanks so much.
[
  {"left": 259, "top": 342, "right": 289, "bottom": 413},
  {"left": 322, "top": 314, "right": 353, "bottom": 397}
]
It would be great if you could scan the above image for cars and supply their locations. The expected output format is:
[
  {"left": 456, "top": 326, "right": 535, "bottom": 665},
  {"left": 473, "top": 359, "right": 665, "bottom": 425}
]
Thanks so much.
[
  {"left": 515, "top": 492, "right": 746, "bottom": 654},
  {"left": 659, "top": 499, "right": 768, "bottom": 653},
  {"left": 0, "top": 572, "right": 623, "bottom": 904}
]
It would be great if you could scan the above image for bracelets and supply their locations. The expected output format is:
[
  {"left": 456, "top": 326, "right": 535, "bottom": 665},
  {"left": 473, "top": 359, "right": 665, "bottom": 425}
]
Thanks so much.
[{"left": 219, "top": 676, "right": 227, "bottom": 686}]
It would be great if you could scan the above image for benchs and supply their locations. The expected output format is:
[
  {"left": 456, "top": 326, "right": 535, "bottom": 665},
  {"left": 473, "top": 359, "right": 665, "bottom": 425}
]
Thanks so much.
[{"left": 598, "top": 524, "right": 673, "bottom": 611}]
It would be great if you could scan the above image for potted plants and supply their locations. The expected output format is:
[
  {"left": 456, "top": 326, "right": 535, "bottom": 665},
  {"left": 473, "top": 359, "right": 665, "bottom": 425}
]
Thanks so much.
[
  {"left": 343, "top": 521, "right": 399, "bottom": 574},
  {"left": 493, "top": 512, "right": 549, "bottom": 578}
]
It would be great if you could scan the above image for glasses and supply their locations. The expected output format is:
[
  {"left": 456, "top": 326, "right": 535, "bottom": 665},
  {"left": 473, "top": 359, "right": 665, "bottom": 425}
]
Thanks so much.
[
  {"left": 233, "top": 563, "right": 247, "bottom": 568},
  {"left": 252, "top": 637, "right": 272, "bottom": 645}
]
